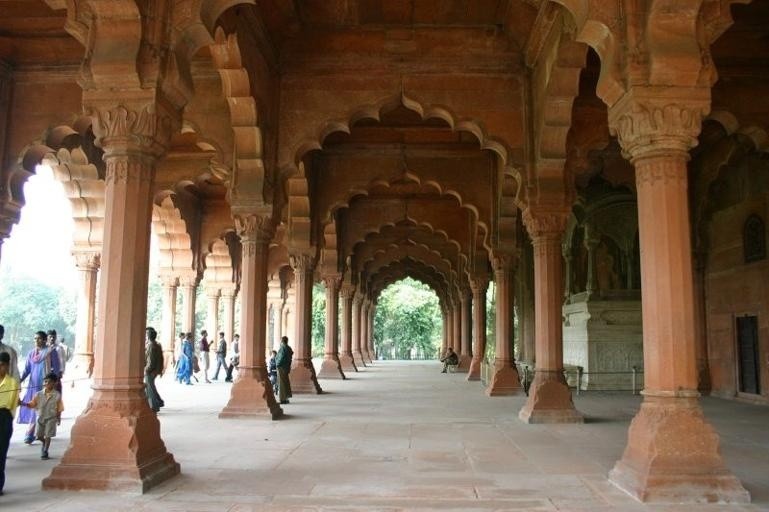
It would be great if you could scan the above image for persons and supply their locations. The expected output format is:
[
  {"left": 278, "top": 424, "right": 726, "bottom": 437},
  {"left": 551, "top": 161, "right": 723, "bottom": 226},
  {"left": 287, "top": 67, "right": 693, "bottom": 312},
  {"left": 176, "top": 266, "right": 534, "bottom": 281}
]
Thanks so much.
[
  {"left": 268, "top": 350, "right": 277, "bottom": 395},
  {"left": 275, "top": 335, "right": 295, "bottom": 404},
  {"left": 440, "top": 347, "right": 459, "bottom": 373},
  {"left": 142, "top": 327, "right": 241, "bottom": 414},
  {"left": 0, "top": 325, "right": 73, "bottom": 497}
]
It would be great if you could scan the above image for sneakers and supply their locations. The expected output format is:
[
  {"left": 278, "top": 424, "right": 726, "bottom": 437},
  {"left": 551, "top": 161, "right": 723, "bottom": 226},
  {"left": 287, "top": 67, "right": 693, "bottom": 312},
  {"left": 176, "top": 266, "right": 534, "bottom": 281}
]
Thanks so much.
[{"left": 40, "top": 447, "right": 49, "bottom": 460}]
